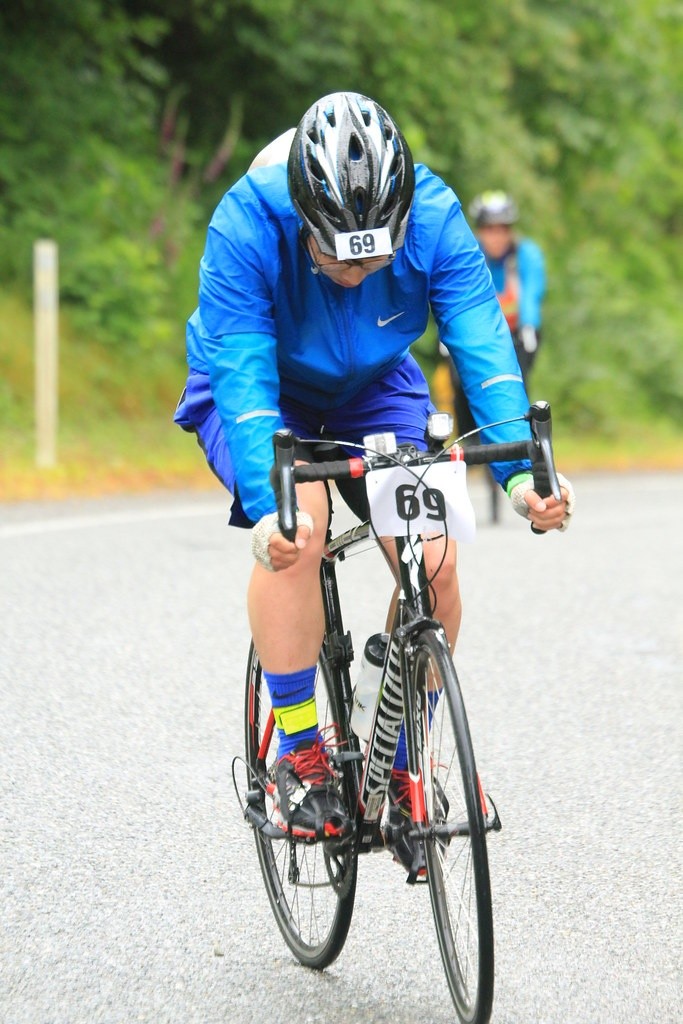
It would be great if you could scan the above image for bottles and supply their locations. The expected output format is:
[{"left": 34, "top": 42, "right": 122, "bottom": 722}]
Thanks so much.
[{"left": 349, "top": 632, "right": 390, "bottom": 743}]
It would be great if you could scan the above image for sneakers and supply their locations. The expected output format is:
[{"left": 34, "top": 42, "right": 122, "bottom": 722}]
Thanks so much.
[
  {"left": 379, "top": 753, "right": 451, "bottom": 876},
  {"left": 264, "top": 723, "right": 353, "bottom": 842}
]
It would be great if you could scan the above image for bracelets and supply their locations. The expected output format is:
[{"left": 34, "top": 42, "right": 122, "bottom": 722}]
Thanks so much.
[{"left": 507, "top": 474, "right": 529, "bottom": 498}]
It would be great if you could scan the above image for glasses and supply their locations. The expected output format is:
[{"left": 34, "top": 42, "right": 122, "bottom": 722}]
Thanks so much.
[{"left": 307, "top": 237, "right": 397, "bottom": 273}]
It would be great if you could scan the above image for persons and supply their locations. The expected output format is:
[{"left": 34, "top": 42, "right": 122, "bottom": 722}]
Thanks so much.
[
  {"left": 174, "top": 91, "right": 573, "bottom": 871},
  {"left": 446, "top": 190, "right": 550, "bottom": 447}
]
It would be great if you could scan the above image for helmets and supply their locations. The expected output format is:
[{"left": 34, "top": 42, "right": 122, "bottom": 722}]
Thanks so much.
[
  {"left": 468, "top": 189, "right": 521, "bottom": 225},
  {"left": 287, "top": 91, "right": 415, "bottom": 261}
]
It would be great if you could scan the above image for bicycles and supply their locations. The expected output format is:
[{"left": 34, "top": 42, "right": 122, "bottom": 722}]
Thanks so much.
[
  {"left": 229, "top": 401, "right": 563, "bottom": 1024},
  {"left": 482, "top": 341, "right": 524, "bottom": 525}
]
